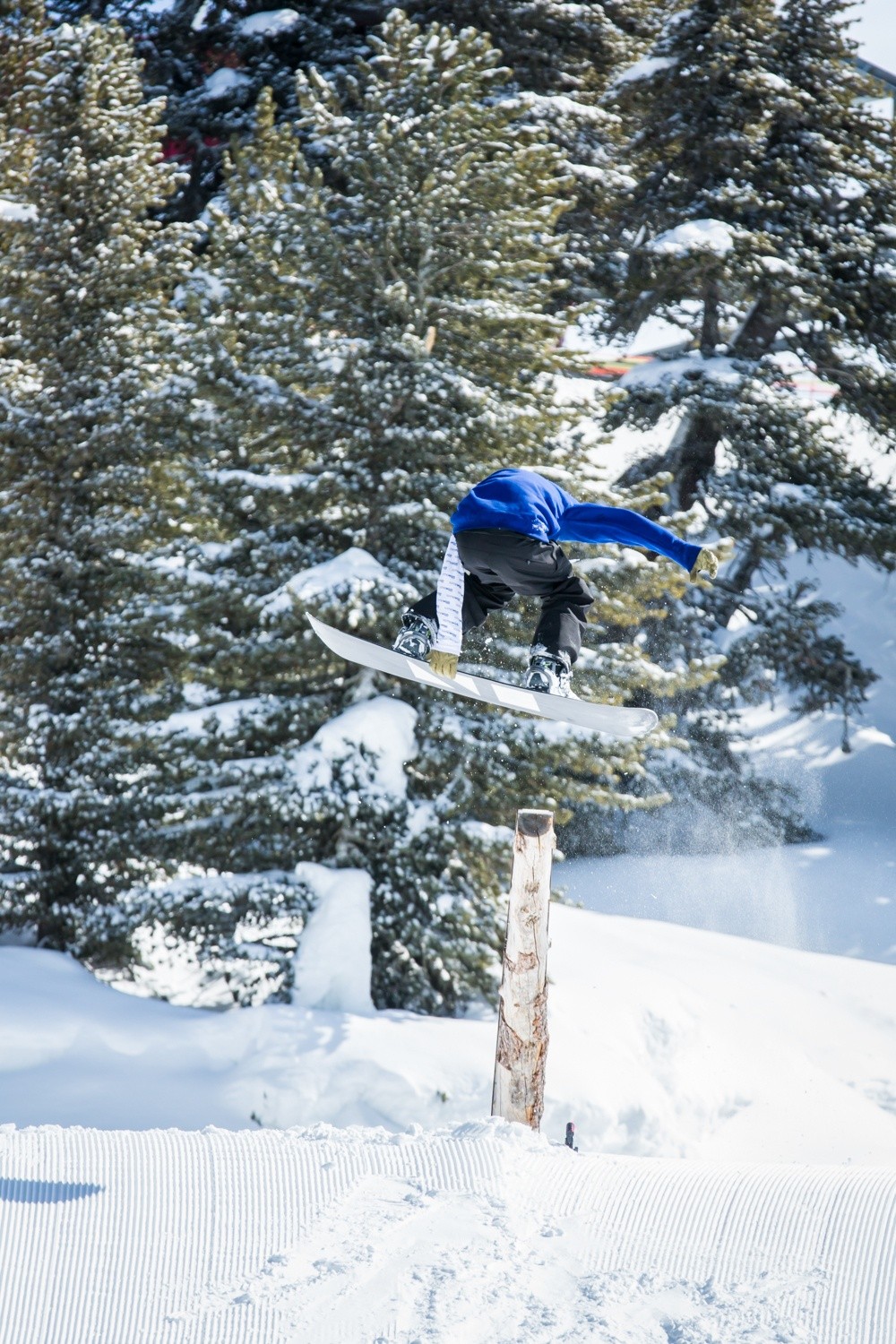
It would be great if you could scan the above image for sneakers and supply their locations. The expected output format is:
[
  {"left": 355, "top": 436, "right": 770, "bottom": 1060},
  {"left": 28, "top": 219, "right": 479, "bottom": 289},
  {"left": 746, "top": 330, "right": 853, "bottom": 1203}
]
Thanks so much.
[
  {"left": 391, "top": 613, "right": 437, "bottom": 662},
  {"left": 526, "top": 651, "right": 582, "bottom": 700}
]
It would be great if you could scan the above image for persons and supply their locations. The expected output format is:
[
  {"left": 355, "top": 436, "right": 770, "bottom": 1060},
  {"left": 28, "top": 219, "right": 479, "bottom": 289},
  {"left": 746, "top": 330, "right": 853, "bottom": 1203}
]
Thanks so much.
[{"left": 393, "top": 467, "right": 737, "bottom": 693}]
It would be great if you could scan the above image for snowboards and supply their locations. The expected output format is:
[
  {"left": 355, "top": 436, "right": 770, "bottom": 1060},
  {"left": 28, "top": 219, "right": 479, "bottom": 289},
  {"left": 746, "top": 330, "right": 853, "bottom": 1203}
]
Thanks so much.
[{"left": 307, "top": 610, "right": 658, "bottom": 742}]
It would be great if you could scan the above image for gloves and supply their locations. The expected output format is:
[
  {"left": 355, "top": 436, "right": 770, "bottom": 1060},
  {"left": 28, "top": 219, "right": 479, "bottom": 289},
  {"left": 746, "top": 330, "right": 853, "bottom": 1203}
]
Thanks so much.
[
  {"left": 690, "top": 536, "right": 734, "bottom": 584},
  {"left": 424, "top": 649, "right": 459, "bottom": 678}
]
